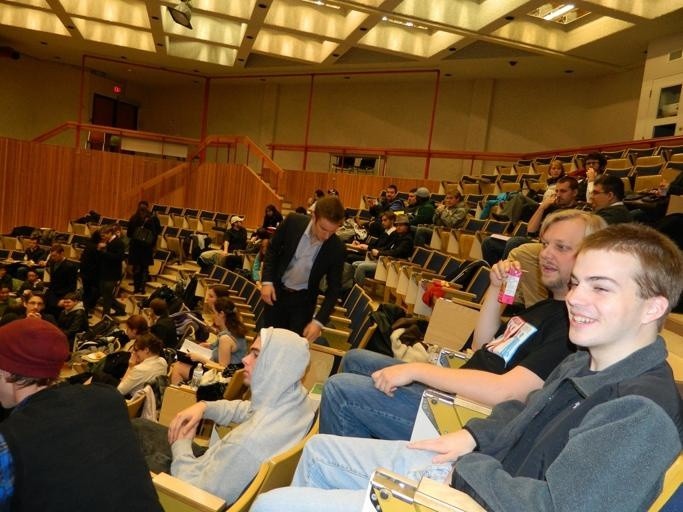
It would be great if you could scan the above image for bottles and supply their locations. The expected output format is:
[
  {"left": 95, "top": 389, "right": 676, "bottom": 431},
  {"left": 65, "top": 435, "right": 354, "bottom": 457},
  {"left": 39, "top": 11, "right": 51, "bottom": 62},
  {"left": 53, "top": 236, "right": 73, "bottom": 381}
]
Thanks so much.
[{"left": 190, "top": 362, "right": 203, "bottom": 391}]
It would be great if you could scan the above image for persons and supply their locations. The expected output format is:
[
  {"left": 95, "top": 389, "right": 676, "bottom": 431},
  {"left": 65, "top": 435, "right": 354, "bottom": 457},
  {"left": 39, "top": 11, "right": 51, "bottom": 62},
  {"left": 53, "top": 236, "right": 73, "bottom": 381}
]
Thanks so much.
[
  {"left": 250, "top": 221, "right": 683, "bottom": 512},
  {"left": 1, "top": 199, "right": 247, "bottom": 422},
  {"left": 481, "top": 154, "right": 624, "bottom": 311},
  {"left": 0, "top": 315, "right": 165, "bottom": 512},
  {"left": 128, "top": 324, "right": 313, "bottom": 509},
  {"left": 318, "top": 210, "right": 608, "bottom": 444},
  {"left": 249, "top": 185, "right": 472, "bottom": 343}
]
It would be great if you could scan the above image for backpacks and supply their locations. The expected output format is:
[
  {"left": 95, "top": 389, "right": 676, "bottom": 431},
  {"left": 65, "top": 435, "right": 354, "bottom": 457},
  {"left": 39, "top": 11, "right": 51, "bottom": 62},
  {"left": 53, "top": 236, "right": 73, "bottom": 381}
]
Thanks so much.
[
  {"left": 443, "top": 258, "right": 493, "bottom": 292},
  {"left": 76, "top": 314, "right": 121, "bottom": 345},
  {"left": 105, "top": 329, "right": 134, "bottom": 350},
  {"left": 72, "top": 336, "right": 122, "bottom": 362},
  {"left": 169, "top": 309, "right": 210, "bottom": 342}
]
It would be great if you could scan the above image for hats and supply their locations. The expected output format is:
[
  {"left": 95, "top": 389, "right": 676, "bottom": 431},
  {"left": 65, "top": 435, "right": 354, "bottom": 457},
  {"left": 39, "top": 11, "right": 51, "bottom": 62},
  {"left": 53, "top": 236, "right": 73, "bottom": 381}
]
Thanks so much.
[
  {"left": 0, "top": 317, "right": 69, "bottom": 380},
  {"left": 414, "top": 187, "right": 431, "bottom": 197},
  {"left": 230, "top": 215, "right": 245, "bottom": 224},
  {"left": 394, "top": 214, "right": 410, "bottom": 224}
]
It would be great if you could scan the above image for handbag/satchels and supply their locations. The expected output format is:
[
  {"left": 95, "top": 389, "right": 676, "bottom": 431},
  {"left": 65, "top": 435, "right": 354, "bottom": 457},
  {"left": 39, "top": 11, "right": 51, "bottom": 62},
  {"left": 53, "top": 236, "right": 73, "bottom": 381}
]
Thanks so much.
[{"left": 133, "top": 225, "right": 154, "bottom": 243}]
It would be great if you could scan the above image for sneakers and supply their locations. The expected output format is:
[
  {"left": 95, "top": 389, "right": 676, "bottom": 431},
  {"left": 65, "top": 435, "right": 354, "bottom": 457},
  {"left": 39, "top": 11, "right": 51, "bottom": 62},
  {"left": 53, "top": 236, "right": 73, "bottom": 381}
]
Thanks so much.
[{"left": 492, "top": 212, "right": 514, "bottom": 234}]
